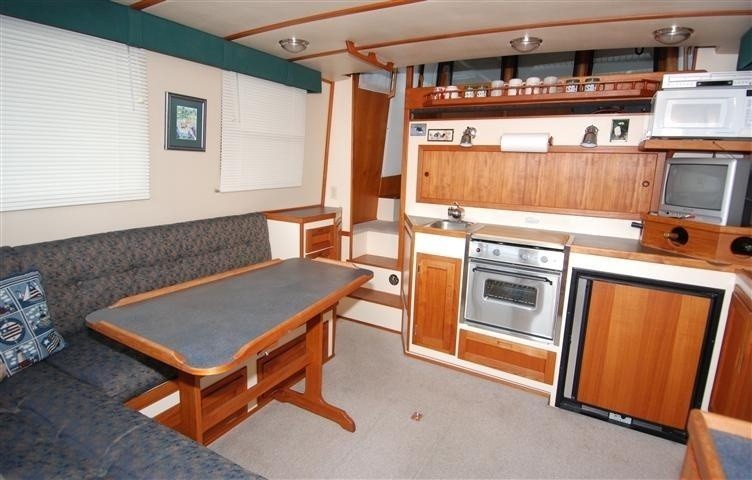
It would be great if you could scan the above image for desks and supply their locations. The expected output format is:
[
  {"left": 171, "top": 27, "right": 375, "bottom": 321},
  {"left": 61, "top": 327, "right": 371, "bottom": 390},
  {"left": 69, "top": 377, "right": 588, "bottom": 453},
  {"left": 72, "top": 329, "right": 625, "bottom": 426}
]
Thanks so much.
[
  {"left": 681, "top": 406, "right": 752, "bottom": 480},
  {"left": 86, "top": 256, "right": 372, "bottom": 447}
]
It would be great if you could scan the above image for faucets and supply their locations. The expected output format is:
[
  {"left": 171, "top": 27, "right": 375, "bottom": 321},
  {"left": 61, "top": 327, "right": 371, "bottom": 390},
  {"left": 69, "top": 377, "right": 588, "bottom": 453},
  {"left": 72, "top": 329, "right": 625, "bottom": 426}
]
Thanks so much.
[{"left": 448, "top": 201, "right": 462, "bottom": 220}]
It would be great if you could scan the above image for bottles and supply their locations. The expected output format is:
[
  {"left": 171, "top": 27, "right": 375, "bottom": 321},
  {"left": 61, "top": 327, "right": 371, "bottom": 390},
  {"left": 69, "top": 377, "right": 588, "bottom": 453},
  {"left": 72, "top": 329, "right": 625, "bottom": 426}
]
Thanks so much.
[{"left": 432, "top": 76, "right": 600, "bottom": 100}]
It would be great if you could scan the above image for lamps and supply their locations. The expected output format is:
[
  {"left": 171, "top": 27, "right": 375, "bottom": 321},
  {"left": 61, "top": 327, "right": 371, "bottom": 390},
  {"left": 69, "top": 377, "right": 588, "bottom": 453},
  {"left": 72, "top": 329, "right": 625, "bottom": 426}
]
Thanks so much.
[
  {"left": 652, "top": 28, "right": 695, "bottom": 45},
  {"left": 581, "top": 125, "right": 599, "bottom": 148},
  {"left": 509, "top": 37, "right": 544, "bottom": 53},
  {"left": 278, "top": 39, "right": 309, "bottom": 54},
  {"left": 460, "top": 126, "right": 476, "bottom": 147}
]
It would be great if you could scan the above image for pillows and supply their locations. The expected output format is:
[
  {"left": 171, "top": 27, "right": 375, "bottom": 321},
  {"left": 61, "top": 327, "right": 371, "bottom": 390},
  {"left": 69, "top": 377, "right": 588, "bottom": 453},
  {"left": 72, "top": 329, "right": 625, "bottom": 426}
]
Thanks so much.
[{"left": 0, "top": 271, "right": 69, "bottom": 384}]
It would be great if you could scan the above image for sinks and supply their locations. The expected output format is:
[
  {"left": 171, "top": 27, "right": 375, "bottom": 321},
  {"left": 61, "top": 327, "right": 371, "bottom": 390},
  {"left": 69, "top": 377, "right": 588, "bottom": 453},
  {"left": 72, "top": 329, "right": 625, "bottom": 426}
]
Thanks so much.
[{"left": 423, "top": 217, "right": 476, "bottom": 232}]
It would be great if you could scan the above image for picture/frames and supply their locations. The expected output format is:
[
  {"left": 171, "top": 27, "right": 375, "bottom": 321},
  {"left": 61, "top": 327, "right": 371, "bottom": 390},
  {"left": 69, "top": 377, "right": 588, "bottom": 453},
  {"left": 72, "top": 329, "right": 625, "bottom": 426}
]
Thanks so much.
[{"left": 164, "top": 91, "right": 208, "bottom": 151}]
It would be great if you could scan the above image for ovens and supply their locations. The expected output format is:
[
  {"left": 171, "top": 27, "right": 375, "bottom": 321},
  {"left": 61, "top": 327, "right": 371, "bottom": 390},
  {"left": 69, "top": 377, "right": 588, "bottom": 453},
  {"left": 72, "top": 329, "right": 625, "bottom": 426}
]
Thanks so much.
[{"left": 461, "top": 258, "right": 567, "bottom": 341}]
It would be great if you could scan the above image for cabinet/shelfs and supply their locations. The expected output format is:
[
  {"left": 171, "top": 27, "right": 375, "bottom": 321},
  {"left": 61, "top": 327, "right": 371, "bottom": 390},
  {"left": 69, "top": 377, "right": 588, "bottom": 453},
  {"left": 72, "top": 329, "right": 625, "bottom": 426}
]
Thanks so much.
[
  {"left": 708, "top": 270, "right": 752, "bottom": 422},
  {"left": 416, "top": 145, "right": 667, "bottom": 220},
  {"left": 401, "top": 228, "right": 468, "bottom": 368},
  {"left": 458, "top": 320, "right": 557, "bottom": 399},
  {"left": 551, "top": 243, "right": 736, "bottom": 446},
  {"left": 260, "top": 206, "right": 341, "bottom": 261}
]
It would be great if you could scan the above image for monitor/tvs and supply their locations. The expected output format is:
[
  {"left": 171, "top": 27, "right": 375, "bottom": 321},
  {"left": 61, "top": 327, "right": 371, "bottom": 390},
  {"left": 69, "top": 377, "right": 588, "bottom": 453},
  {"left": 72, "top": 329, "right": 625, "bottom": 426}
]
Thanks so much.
[{"left": 657, "top": 158, "right": 752, "bottom": 227}]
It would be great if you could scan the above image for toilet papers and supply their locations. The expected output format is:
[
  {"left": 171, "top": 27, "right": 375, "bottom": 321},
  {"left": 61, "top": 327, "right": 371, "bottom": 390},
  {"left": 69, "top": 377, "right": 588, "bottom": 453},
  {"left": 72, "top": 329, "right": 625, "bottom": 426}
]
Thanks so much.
[{"left": 499, "top": 132, "right": 552, "bottom": 153}]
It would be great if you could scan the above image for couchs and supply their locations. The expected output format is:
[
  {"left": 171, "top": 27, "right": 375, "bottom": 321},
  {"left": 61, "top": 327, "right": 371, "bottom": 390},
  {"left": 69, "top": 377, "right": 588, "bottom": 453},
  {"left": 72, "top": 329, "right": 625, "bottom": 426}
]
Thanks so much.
[{"left": 1, "top": 213, "right": 264, "bottom": 480}]
